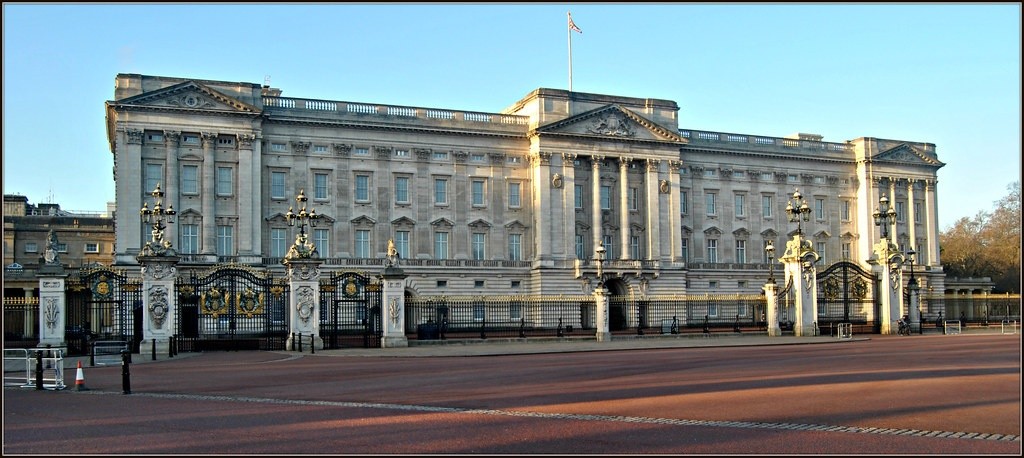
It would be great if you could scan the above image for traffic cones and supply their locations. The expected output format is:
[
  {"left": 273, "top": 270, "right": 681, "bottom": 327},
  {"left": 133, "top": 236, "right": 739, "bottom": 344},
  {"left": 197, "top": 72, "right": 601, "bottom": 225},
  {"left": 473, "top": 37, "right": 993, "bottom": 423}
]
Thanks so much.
[{"left": 73, "top": 360, "right": 86, "bottom": 390}]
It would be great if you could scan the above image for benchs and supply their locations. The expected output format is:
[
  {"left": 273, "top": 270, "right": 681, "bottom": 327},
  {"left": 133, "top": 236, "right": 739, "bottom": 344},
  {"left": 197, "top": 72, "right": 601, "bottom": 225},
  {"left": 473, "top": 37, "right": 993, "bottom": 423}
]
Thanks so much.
[{"left": 93, "top": 340, "right": 127, "bottom": 356}]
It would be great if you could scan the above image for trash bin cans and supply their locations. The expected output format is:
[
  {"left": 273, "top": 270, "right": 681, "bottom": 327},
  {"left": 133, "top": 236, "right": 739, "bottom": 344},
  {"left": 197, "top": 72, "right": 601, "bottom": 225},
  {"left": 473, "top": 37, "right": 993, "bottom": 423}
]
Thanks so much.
[{"left": 37, "top": 342, "right": 51, "bottom": 369}]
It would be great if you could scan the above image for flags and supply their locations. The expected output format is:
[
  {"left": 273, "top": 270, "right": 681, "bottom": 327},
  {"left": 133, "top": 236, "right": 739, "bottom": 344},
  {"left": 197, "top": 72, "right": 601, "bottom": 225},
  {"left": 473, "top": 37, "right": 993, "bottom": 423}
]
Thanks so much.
[{"left": 569, "top": 17, "right": 582, "bottom": 33}]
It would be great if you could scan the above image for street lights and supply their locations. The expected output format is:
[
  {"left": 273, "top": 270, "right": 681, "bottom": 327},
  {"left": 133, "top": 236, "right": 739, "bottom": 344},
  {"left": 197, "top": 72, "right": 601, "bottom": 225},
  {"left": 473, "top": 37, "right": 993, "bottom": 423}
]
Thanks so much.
[
  {"left": 765, "top": 240, "right": 777, "bottom": 284},
  {"left": 282, "top": 189, "right": 316, "bottom": 244},
  {"left": 595, "top": 241, "right": 607, "bottom": 284},
  {"left": 141, "top": 184, "right": 177, "bottom": 243},
  {"left": 874, "top": 192, "right": 899, "bottom": 244},
  {"left": 785, "top": 188, "right": 812, "bottom": 234}
]
